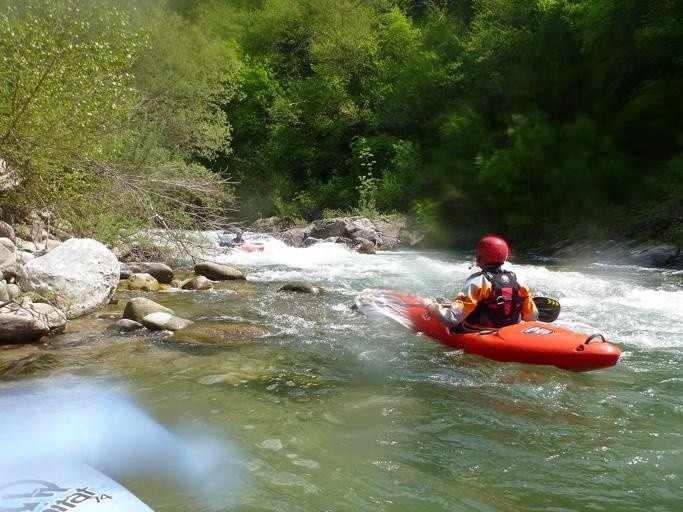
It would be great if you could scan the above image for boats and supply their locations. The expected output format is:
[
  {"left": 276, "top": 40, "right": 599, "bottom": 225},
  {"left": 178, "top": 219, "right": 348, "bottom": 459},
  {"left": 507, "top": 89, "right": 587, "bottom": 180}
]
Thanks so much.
[
  {"left": 218, "top": 241, "right": 264, "bottom": 254},
  {"left": 381, "top": 287, "right": 620, "bottom": 376}
]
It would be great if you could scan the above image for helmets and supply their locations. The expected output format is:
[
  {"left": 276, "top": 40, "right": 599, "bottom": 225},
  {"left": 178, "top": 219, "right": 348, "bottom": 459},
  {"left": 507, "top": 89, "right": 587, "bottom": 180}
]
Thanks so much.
[{"left": 477, "top": 236, "right": 508, "bottom": 266}]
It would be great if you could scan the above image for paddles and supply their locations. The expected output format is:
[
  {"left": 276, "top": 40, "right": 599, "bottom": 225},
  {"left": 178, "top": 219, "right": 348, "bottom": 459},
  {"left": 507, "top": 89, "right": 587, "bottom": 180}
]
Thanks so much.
[{"left": 399, "top": 297, "right": 561, "bottom": 322}]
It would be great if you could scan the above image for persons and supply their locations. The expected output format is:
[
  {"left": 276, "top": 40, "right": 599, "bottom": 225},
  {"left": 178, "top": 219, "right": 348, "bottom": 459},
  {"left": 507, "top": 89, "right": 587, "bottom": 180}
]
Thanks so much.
[
  {"left": 420, "top": 236, "right": 539, "bottom": 334},
  {"left": 232, "top": 231, "right": 245, "bottom": 243}
]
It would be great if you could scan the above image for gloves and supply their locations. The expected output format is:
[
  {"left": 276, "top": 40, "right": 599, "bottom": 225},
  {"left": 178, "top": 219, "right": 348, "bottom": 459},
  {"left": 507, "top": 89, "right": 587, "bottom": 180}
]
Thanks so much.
[{"left": 421, "top": 299, "right": 433, "bottom": 307}]
